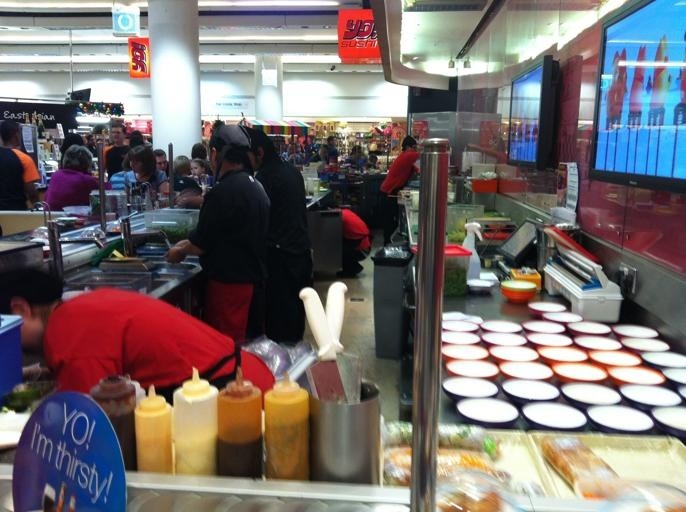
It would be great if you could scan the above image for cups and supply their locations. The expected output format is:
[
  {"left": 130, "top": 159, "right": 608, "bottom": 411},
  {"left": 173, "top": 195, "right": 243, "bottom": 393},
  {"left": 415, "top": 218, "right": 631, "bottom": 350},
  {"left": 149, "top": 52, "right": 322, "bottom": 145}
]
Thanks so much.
[{"left": 306, "top": 377, "right": 383, "bottom": 487}]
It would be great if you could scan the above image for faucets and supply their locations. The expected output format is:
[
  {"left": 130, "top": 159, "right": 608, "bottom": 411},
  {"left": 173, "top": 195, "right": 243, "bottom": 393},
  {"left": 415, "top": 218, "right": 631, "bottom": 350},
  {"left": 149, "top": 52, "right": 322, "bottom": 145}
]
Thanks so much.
[
  {"left": 31, "top": 201, "right": 48, "bottom": 227},
  {"left": 119, "top": 210, "right": 168, "bottom": 257},
  {"left": 49, "top": 221, "right": 104, "bottom": 282},
  {"left": 37, "top": 200, "right": 52, "bottom": 220}
]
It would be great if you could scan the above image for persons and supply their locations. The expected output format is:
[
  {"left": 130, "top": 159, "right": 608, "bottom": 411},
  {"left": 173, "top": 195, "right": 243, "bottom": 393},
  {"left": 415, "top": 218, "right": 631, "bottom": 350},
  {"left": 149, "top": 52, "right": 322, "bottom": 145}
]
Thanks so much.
[{"left": 1, "top": 118, "right": 424, "bottom": 410}]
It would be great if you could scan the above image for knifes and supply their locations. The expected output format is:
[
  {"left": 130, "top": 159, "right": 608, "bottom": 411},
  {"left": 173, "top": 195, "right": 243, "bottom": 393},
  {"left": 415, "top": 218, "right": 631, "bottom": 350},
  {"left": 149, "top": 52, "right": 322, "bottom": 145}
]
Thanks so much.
[
  {"left": 325, "top": 279, "right": 362, "bottom": 402},
  {"left": 299, "top": 287, "right": 347, "bottom": 404}
]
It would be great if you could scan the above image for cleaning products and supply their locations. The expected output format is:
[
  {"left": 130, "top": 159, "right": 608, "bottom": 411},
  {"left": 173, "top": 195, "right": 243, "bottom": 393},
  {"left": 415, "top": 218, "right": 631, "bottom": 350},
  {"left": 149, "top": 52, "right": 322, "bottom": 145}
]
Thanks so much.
[{"left": 461, "top": 222, "right": 484, "bottom": 281}]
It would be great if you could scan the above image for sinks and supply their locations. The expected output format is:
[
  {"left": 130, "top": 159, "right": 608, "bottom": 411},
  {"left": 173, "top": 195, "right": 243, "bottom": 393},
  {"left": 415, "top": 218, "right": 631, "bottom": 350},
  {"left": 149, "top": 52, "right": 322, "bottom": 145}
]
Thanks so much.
[
  {"left": 136, "top": 254, "right": 200, "bottom": 275},
  {"left": 65, "top": 269, "right": 181, "bottom": 297}
]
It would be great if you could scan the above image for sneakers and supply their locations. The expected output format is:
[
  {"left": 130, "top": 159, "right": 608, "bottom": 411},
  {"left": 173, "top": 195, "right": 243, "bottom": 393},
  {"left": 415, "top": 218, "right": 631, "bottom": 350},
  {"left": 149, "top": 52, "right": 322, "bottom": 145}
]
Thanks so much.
[{"left": 336, "top": 263, "right": 363, "bottom": 278}]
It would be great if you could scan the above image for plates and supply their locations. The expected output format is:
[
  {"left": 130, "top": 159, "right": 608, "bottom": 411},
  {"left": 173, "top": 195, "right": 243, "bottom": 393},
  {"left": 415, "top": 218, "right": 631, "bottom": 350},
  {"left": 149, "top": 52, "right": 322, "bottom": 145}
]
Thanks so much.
[{"left": 463, "top": 277, "right": 495, "bottom": 293}]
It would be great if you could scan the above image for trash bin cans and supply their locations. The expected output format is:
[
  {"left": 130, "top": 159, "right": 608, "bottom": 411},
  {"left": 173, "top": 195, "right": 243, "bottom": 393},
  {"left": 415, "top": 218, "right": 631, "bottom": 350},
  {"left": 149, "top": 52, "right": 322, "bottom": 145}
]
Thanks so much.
[{"left": 370, "top": 246, "right": 414, "bottom": 360}]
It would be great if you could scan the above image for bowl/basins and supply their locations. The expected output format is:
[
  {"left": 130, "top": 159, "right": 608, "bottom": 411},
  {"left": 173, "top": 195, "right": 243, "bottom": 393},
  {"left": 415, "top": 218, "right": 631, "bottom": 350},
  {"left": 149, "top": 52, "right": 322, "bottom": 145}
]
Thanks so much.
[
  {"left": 455, "top": 396, "right": 520, "bottom": 428},
  {"left": 499, "top": 279, "right": 537, "bottom": 304},
  {"left": 586, "top": 406, "right": 655, "bottom": 434},
  {"left": 440, "top": 311, "right": 686, "bottom": 413},
  {"left": 28, "top": 235, "right": 51, "bottom": 259},
  {"left": 521, "top": 398, "right": 588, "bottom": 430},
  {"left": 526, "top": 301, "right": 567, "bottom": 316},
  {"left": 649, "top": 406, "right": 686, "bottom": 441}
]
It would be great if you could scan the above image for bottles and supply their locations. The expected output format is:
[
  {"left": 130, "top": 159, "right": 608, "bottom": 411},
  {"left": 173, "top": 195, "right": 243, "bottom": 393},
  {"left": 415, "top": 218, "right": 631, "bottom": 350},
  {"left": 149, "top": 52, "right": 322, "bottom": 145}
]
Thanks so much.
[
  {"left": 124, "top": 373, "right": 145, "bottom": 404},
  {"left": 311, "top": 178, "right": 320, "bottom": 197},
  {"left": 263, "top": 373, "right": 312, "bottom": 482},
  {"left": 216, "top": 365, "right": 266, "bottom": 481},
  {"left": 304, "top": 176, "right": 312, "bottom": 199},
  {"left": 132, "top": 382, "right": 174, "bottom": 473},
  {"left": 172, "top": 365, "right": 218, "bottom": 478},
  {"left": 89, "top": 358, "right": 135, "bottom": 473}
]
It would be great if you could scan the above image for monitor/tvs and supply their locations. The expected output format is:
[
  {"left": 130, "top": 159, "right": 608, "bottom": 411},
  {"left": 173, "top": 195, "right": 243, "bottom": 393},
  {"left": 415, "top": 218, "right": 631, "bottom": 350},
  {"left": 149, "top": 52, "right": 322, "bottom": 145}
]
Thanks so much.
[
  {"left": 506, "top": 56, "right": 562, "bottom": 171},
  {"left": 497, "top": 215, "right": 542, "bottom": 265},
  {"left": 587, "top": 0, "right": 686, "bottom": 193}
]
